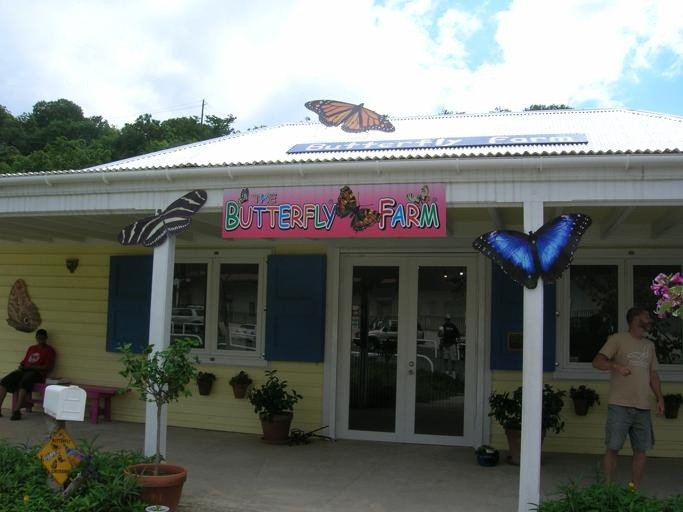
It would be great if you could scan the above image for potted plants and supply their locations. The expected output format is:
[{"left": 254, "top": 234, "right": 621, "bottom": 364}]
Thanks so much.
[
  {"left": 229, "top": 371, "right": 252, "bottom": 398},
  {"left": 244, "top": 367, "right": 304, "bottom": 443},
  {"left": 568, "top": 383, "right": 601, "bottom": 417},
  {"left": 116, "top": 333, "right": 203, "bottom": 510},
  {"left": 659, "top": 390, "right": 681, "bottom": 419},
  {"left": 485, "top": 381, "right": 564, "bottom": 467},
  {"left": 144, "top": 504, "right": 169, "bottom": 512},
  {"left": 196, "top": 371, "right": 214, "bottom": 396}
]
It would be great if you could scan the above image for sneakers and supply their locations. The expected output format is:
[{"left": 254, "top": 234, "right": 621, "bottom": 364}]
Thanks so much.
[
  {"left": 10, "top": 411, "right": 20, "bottom": 420},
  {"left": 449, "top": 374, "right": 455, "bottom": 380},
  {"left": 440, "top": 370, "right": 449, "bottom": 375}
]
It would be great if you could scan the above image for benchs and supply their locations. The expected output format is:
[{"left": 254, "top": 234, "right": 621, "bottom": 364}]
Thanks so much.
[{"left": 8, "top": 377, "right": 131, "bottom": 424}]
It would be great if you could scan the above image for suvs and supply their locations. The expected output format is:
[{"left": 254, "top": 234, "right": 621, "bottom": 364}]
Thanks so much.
[
  {"left": 170, "top": 303, "right": 207, "bottom": 330},
  {"left": 352, "top": 317, "right": 427, "bottom": 354}
]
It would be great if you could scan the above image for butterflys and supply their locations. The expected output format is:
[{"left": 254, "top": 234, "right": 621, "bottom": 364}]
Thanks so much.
[
  {"left": 331, "top": 185, "right": 383, "bottom": 232},
  {"left": 239, "top": 187, "right": 250, "bottom": 204},
  {"left": 116, "top": 189, "right": 208, "bottom": 249},
  {"left": 472, "top": 212, "right": 593, "bottom": 290},
  {"left": 406, "top": 184, "right": 431, "bottom": 204},
  {"left": 4, "top": 278, "right": 42, "bottom": 333},
  {"left": 303, "top": 99, "right": 396, "bottom": 133},
  {"left": 361, "top": 210, "right": 365, "bottom": 212},
  {"left": 181, "top": 221, "right": 183, "bottom": 224}
]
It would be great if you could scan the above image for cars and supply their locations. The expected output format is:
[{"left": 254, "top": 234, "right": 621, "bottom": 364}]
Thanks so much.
[{"left": 229, "top": 320, "right": 257, "bottom": 345}]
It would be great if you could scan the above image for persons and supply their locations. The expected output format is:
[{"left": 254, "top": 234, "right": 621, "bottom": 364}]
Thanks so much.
[
  {"left": 438, "top": 315, "right": 459, "bottom": 380},
  {"left": 591, "top": 308, "right": 665, "bottom": 491},
  {"left": 0, "top": 329, "right": 55, "bottom": 420}
]
